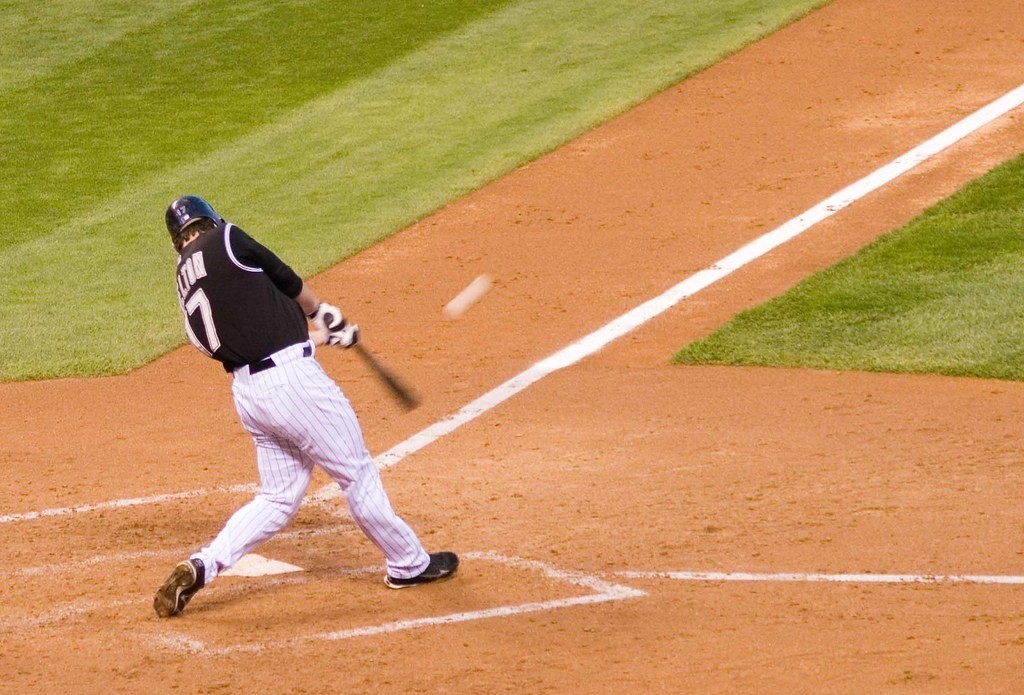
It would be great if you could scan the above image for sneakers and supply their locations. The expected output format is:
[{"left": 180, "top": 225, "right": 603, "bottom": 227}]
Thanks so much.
[
  {"left": 383, "top": 552, "right": 458, "bottom": 589},
  {"left": 152, "top": 558, "right": 206, "bottom": 619}
]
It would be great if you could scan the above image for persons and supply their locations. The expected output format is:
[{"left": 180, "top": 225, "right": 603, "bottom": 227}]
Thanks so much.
[{"left": 154, "top": 195, "right": 458, "bottom": 618}]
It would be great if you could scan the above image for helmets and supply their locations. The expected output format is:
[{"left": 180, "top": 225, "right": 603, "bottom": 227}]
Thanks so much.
[{"left": 165, "top": 196, "right": 227, "bottom": 238}]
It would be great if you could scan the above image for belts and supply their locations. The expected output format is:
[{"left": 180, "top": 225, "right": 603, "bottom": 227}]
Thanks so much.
[{"left": 231, "top": 346, "right": 312, "bottom": 379}]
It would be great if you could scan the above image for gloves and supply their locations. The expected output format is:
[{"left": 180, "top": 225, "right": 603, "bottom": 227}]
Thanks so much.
[{"left": 307, "top": 302, "right": 359, "bottom": 349}]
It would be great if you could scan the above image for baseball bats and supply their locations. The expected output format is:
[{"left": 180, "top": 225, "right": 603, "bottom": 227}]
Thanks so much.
[{"left": 324, "top": 310, "right": 424, "bottom": 415}]
